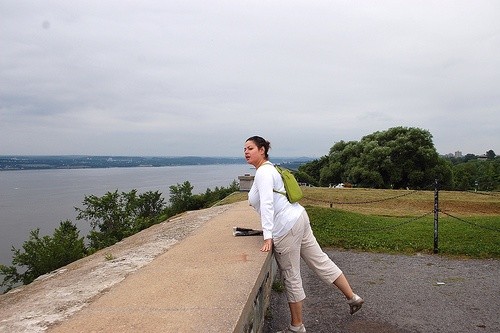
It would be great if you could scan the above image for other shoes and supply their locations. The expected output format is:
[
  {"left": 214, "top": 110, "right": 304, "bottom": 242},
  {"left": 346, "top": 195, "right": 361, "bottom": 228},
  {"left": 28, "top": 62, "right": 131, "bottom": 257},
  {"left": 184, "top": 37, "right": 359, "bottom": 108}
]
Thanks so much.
[
  {"left": 347, "top": 294, "right": 364, "bottom": 314},
  {"left": 277, "top": 324, "right": 306, "bottom": 333}
]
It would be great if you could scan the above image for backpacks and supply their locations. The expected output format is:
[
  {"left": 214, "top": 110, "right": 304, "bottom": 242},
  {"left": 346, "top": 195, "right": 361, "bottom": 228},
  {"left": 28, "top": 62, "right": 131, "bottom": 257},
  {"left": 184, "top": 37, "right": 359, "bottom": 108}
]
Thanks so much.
[{"left": 261, "top": 163, "right": 304, "bottom": 204}]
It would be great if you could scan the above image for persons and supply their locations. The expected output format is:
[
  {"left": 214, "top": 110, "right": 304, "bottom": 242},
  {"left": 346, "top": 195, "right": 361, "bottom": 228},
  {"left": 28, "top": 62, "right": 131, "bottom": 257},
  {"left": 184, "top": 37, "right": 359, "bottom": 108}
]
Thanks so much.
[{"left": 244, "top": 136, "right": 366, "bottom": 333}]
